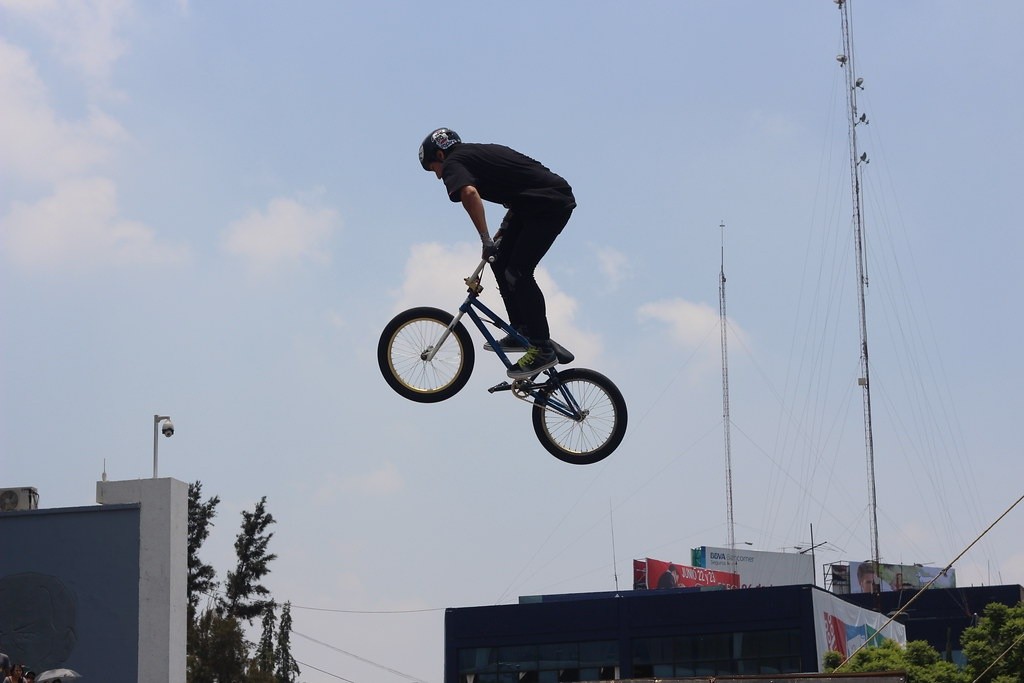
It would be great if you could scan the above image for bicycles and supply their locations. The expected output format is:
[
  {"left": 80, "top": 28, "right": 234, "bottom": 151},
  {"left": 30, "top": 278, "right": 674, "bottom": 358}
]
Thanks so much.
[{"left": 376, "top": 237, "right": 628, "bottom": 466}]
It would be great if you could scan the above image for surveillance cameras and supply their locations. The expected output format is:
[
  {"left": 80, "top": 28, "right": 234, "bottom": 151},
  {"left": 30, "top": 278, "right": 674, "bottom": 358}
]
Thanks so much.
[{"left": 162, "top": 420, "right": 174, "bottom": 437}]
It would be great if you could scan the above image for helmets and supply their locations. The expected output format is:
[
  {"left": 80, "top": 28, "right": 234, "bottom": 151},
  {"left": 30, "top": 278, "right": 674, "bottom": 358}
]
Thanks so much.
[{"left": 419, "top": 128, "right": 461, "bottom": 171}]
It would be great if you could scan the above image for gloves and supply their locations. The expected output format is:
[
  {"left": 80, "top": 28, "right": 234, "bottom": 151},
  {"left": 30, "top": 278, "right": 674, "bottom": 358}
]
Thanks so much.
[
  {"left": 481, "top": 230, "right": 498, "bottom": 263},
  {"left": 499, "top": 211, "right": 513, "bottom": 235}
]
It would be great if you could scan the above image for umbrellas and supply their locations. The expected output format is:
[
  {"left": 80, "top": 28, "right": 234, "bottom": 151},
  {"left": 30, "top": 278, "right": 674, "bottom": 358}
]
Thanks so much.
[{"left": 34, "top": 668, "right": 82, "bottom": 683}]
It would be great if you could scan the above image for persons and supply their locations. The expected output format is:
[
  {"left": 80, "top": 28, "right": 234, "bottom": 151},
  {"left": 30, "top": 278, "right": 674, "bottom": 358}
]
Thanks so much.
[
  {"left": 657, "top": 564, "right": 680, "bottom": 589},
  {"left": 857, "top": 560, "right": 877, "bottom": 594},
  {"left": 0, "top": 652, "right": 63, "bottom": 683},
  {"left": 891, "top": 573, "right": 902, "bottom": 590},
  {"left": 419, "top": 127, "right": 576, "bottom": 378}
]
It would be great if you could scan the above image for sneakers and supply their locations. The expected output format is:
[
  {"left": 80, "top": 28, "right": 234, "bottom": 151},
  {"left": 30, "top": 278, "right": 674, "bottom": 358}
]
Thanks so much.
[
  {"left": 483, "top": 329, "right": 530, "bottom": 352},
  {"left": 507, "top": 342, "right": 559, "bottom": 378}
]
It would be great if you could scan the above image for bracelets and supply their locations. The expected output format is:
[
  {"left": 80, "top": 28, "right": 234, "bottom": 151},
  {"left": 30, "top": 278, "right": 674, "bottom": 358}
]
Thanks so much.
[{"left": 480, "top": 230, "right": 489, "bottom": 237}]
